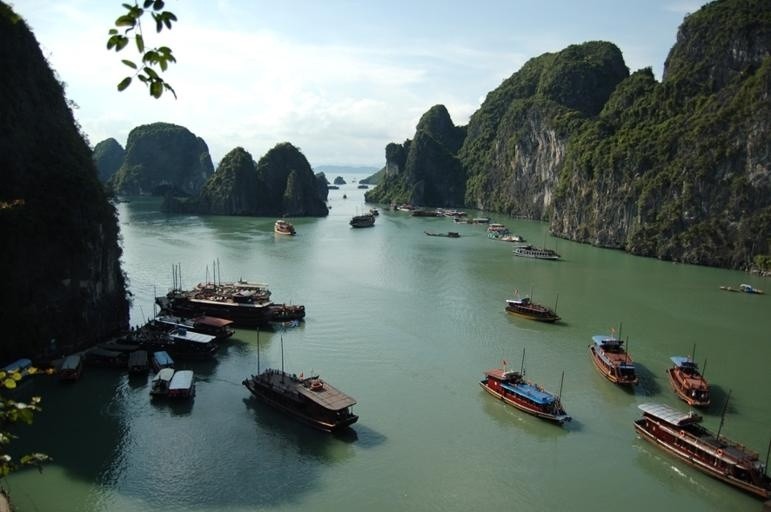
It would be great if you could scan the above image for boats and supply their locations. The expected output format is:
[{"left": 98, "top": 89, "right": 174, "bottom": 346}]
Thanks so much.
[
  {"left": 242, "top": 323, "right": 361, "bottom": 432},
  {"left": 50, "top": 256, "right": 307, "bottom": 402},
  {"left": 479, "top": 345, "right": 574, "bottom": 425},
  {"left": 666, "top": 341, "right": 713, "bottom": 406},
  {"left": 718, "top": 279, "right": 766, "bottom": 297},
  {"left": 587, "top": 320, "right": 642, "bottom": 387},
  {"left": 271, "top": 216, "right": 298, "bottom": 240},
  {"left": 346, "top": 202, "right": 563, "bottom": 263},
  {"left": 1, "top": 357, "right": 34, "bottom": 390},
  {"left": 505, "top": 285, "right": 565, "bottom": 325},
  {"left": 633, "top": 402, "right": 771, "bottom": 499}
]
